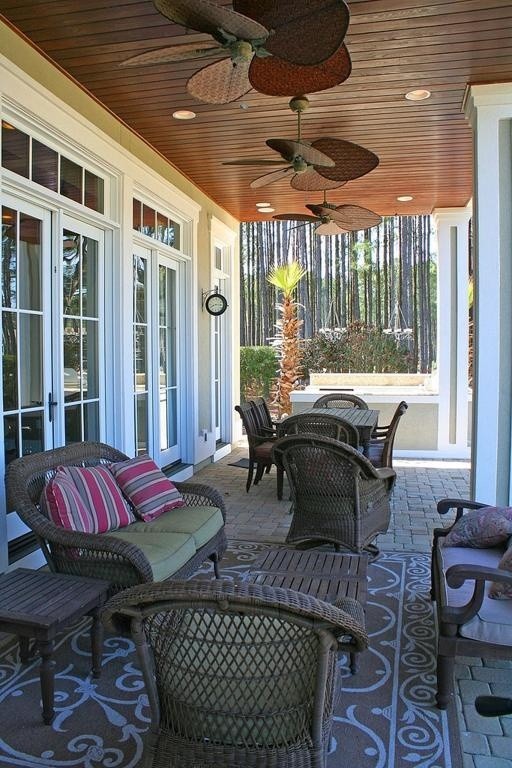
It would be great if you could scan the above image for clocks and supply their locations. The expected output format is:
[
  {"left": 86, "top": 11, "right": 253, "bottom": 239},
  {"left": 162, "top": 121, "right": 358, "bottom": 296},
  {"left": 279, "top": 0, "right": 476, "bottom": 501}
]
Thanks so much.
[{"left": 201, "top": 284, "right": 226, "bottom": 317}]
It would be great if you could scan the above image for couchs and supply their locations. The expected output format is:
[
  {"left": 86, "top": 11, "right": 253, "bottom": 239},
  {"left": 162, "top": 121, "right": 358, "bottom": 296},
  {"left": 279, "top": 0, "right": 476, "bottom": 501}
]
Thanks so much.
[{"left": 5, "top": 442, "right": 227, "bottom": 594}]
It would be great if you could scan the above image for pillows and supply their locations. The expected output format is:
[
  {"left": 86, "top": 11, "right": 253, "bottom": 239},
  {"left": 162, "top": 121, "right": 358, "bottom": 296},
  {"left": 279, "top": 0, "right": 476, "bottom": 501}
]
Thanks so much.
[
  {"left": 58, "top": 463, "right": 138, "bottom": 535},
  {"left": 108, "top": 453, "right": 183, "bottom": 520},
  {"left": 491, "top": 543, "right": 512, "bottom": 599},
  {"left": 443, "top": 505, "right": 511, "bottom": 545},
  {"left": 39, "top": 471, "right": 95, "bottom": 537}
]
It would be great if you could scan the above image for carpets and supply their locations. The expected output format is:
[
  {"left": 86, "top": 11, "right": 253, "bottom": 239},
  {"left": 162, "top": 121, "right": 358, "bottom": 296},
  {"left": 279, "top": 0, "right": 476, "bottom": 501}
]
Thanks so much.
[{"left": 0, "top": 533, "right": 465, "bottom": 766}]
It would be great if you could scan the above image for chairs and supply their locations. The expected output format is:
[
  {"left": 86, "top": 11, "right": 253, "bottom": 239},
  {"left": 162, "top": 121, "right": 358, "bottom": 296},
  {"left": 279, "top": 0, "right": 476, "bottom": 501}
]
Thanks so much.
[
  {"left": 268, "top": 434, "right": 398, "bottom": 565},
  {"left": 249, "top": 396, "right": 280, "bottom": 472},
  {"left": 312, "top": 392, "right": 368, "bottom": 456},
  {"left": 429, "top": 495, "right": 512, "bottom": 725},
  {"left": 105, "top": 577, "right": 367, "bottom": 763},
  {"left": 369, "top": 404, "right": 405, "bottom": 484},
  {"left": 375, "top": 399, "right": 409, "bottom": 481},
  {"left": 234, "top": 402, "right": 281, "bottom": 490},
  {"left": 276, "top": 415, "right": 363, "bottom": 500}
]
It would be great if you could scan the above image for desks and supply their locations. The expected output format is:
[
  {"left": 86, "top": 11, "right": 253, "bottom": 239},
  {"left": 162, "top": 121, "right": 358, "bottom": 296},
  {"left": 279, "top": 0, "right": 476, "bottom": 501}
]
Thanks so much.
[
  {"left": 0, "top": 567, "right": 104, "bottom": 718},
  {"left": 273, "top": 406, "right": 382, "bottom": 504}
]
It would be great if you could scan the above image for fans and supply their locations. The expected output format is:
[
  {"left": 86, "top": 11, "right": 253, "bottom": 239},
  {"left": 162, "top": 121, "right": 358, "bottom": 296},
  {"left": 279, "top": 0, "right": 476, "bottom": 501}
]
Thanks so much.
[
  {"left": 122, "top": 4, "right": 360, "bottom": 104},
  {"left": 271, "top": 189, "right": 384, "bottom": 241},
  {"left": 220, "top": 97, "right": 381, "bottom": 192}
]
